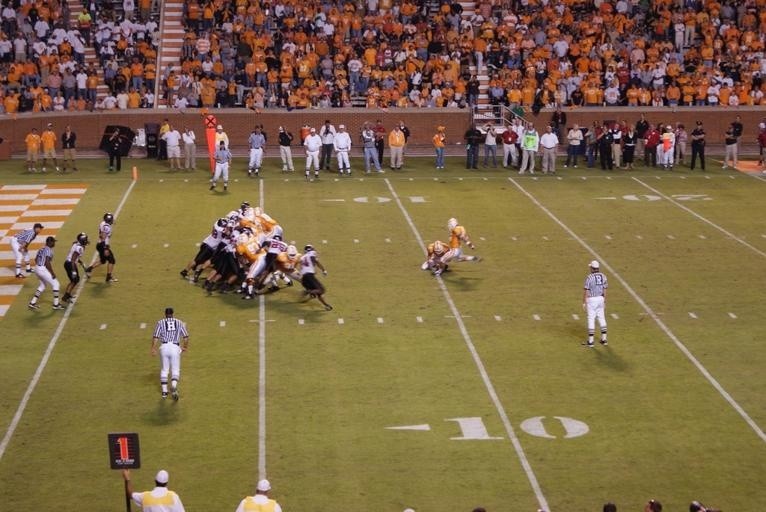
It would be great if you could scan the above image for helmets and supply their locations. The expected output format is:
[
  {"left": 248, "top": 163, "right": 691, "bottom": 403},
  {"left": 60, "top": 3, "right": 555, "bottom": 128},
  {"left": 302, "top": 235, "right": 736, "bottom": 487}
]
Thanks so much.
[
  {"left": 304, "top": 245, "right": 313, "bottom": 252},
  {"left": 77, "top": 233, "right": 88, "bottom": 245},
  {"left": 218, "top": 202, "right": 263, "bottom": 243},
  {"left": 448, "top": 218, "right": 457, "bottom": 231},
  {"left": 104, "top": 213, "right": 114, "bottom": 223},
  {"left": 287, "top": 245, "right": 297, "bottom": 260},
  {"left": 434, "top": 241, "right": 444, "bottom": 255}
]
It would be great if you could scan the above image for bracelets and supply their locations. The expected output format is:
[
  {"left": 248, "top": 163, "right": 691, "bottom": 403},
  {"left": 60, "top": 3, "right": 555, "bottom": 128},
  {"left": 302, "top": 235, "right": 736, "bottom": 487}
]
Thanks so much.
[{"left": 127, "top": 479, "right": 131, "bottom": 481}]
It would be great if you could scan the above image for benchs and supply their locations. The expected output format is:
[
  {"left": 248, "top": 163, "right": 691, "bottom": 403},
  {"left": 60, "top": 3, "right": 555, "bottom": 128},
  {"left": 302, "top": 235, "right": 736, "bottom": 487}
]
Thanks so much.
[{"left": 0, "top": 0, "right": 766, "bottom": 111}]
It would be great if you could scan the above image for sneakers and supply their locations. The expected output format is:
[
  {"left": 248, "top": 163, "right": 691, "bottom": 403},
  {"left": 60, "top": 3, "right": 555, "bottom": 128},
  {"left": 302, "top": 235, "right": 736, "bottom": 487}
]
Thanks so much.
[
  {"left": 582, "top": 341, "right": 593, "bottom": 347},
  {"left": 600, "top": 339, "right": 608, "bottom": 345}
]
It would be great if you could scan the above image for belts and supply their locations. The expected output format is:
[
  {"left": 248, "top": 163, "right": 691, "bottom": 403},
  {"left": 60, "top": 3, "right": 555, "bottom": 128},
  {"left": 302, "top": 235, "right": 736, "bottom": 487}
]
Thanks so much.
[{"left": 162, "top": 342, "right": 180, "bottom": 346}]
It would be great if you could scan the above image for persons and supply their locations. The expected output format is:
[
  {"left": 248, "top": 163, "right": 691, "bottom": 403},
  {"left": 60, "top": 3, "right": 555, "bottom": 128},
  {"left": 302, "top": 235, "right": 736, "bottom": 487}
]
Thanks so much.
[
  {"left": 603, "top": 502, "right": 618, "bottom": 511},
  {"left": 180, "top": 200, "right": 333, "bottom": 312},
  {"left": 464, "top": 118, "right": 560, "bottom": 173},
  {"left": 123, "top": 470, "right": 187, "bottom": 511},
  {"left": 756, "top": 122, "right": 766, "bottom": 168},
  {"left": 234, "top": 479, "right": 284, "bottom": 511},
  {"left": 563, "top": 123, "right": 584, "bottom": 168},
  {"left": 690, "top": 501, "right": 706, "bottom": 512},
  {"left": 730, "top": 115, "right": 744, "bottom": 155},
  {"left": 1, "top": 1, "right": 160, "bottom": 115},
  {"left": 721, "top": 124, "right": 738, "bottom": 167},
  {"left": 108, "top": 128, "right": 123, "bottom": 171},
  {"left": 433, "top": 217, "right": 479, "bottom": 277},
  {"left": 583, "top": 113, "right": 688, "bottom": 171},
  {"left": 279, "top": 120, "right": 352, "bottom": 173},
  {"left": 584, "top": 260, "right": 609, "bottom": 347},
  {"left": 361, "top": 120, "right": 410, "bottom": 173},
  {"left": 61, "top": 232, "right": 90, "bottom": 304},
  {"left": 421, "top": 239, "right": 452, "bottom": 270},
  {"left": 209, "top": 140, "right": 234, "bottom": 190},
  {"left": 151, "top": 308, "right": 188, "bottom": 400},
  {"left": 88, "top": 212, "right": 119, "bottom": 283},
  {"left": 215, "top": 123, "right": 268, "bottom": 173},
  {"left": 691, "top": 120, "right": 706, "bottom": 170},
  {"left": 163, "top": 1, "right": 766, "bottom": 107},
  {"left": 25, "top": 125, "right": 77, "bottom": 172},
  {"left": 10, "top": 224, "right": 42, "bottom": 278},
  {"left": 155, "top": 118, "right": 198, "bottom": 170},
  {"left": 552, "top": 106, "right": 567, "bottom": 144},
  {"left": 433, "top": 125, "right": 447, "bottom": 169},
  {"left": 644, "top": 498, "right": 663, "bottom": 511},
  {"left": 26, "top": 237, "right": 66, "bottom": 311}
]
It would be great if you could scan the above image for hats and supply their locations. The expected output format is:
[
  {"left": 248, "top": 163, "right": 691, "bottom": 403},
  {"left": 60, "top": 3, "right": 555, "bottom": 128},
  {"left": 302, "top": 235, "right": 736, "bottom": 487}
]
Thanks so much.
[
  {"left": 257, "top": 480, "right": 272, "bottom": 491},
  {"left": 156, "top": 470, "right": 169, "bottom": 483},
  {"left": 589, "top": 261, "right": 600, "bottom": 268},
  {"left": 46, "top": 237, "right": 57, "bottom": 242},
  {"left": 34, "top": 224, "right": 43, "bottom": 228},
  {"left": 166, "top": 308, "right": 173, "bottom": 314}
]
